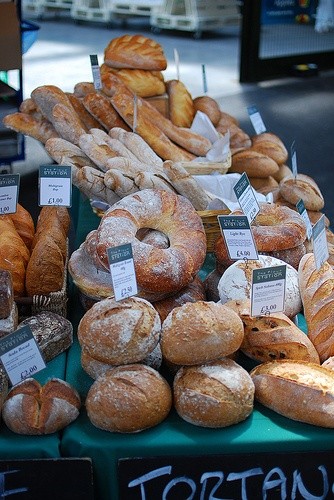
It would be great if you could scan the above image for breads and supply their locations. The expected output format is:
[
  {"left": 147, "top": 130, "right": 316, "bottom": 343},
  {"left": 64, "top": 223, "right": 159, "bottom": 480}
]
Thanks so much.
[{"left": 0, "top": 36, "right": 334, "bottom": 433}]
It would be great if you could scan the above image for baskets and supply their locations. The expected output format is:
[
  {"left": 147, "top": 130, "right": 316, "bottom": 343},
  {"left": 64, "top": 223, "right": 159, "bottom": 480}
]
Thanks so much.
[
  {"left": 175, "top": 132, "right": 232, "bottom": 175},
  {"left": 0, "top": 237, "right": 69, "bottom": 311},
  {"left": 195, "top": 209, "right": 231, "bottom": 253}
]
